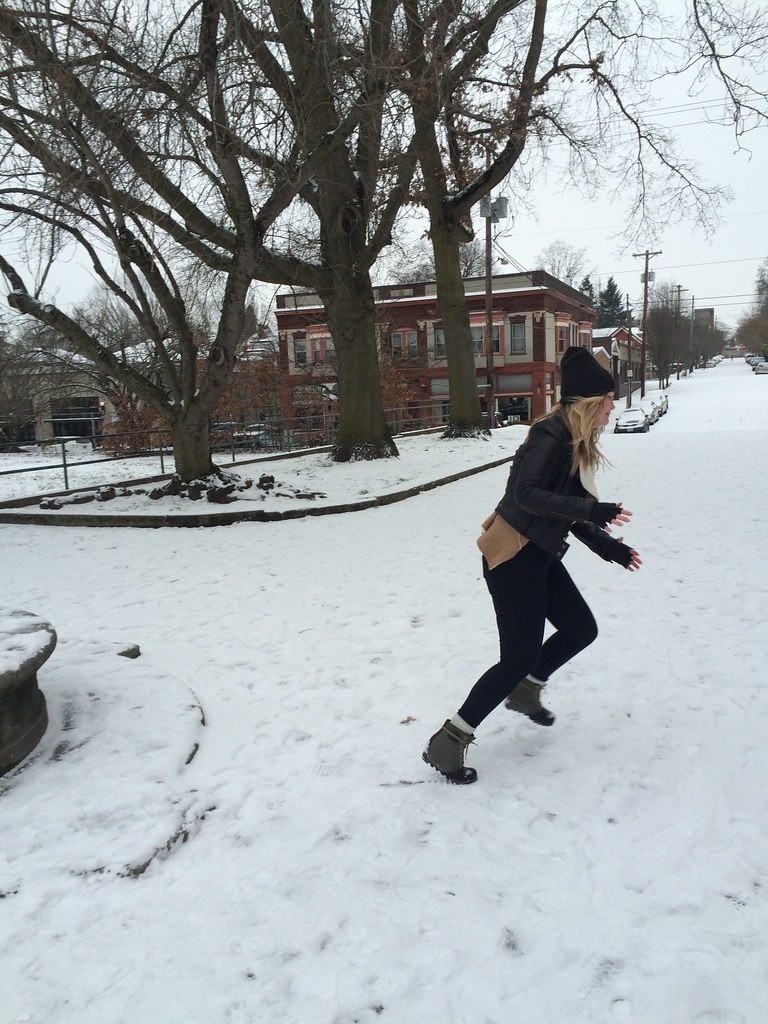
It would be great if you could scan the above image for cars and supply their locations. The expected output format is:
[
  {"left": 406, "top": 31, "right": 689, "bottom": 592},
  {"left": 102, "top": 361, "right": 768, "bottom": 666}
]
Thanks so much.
[
  {"left": 705, "top": 354, "right": 725, "bottom": 367},
  {"left": 614, "top": 389, "right": 669, "bottom": 434},
  {"left": 744, "top": 353, "right": 768, "bottom": 374}
]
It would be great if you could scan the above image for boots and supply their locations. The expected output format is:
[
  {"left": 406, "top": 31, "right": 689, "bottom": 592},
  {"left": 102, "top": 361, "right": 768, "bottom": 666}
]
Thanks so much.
[
  {"left": 422, "top": 719, "right": 479, "bottom": 784},
  {"left": 508, "top": 677, "right": 555, "bottom": 726}
]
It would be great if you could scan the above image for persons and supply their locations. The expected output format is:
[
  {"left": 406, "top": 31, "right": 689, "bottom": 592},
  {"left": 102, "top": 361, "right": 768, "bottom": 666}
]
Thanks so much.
[{"left": 422, "top": 346, "right": 642, "bottom": 784}]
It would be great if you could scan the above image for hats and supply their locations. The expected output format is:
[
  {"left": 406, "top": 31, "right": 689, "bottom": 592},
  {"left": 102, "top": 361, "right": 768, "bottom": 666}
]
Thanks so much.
[{"left": 561, "top": 346, "right": 615, "bottom": 404}]
